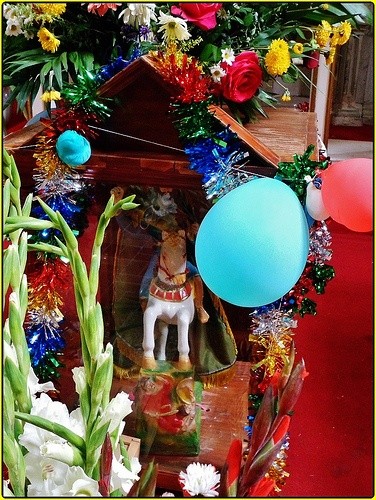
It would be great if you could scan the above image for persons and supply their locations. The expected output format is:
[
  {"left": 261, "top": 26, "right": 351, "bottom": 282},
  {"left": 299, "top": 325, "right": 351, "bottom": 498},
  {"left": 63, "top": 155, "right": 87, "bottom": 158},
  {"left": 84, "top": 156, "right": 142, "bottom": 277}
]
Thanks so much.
[{"left": 128, "top": 185, "right": 209, "bottom": 325}]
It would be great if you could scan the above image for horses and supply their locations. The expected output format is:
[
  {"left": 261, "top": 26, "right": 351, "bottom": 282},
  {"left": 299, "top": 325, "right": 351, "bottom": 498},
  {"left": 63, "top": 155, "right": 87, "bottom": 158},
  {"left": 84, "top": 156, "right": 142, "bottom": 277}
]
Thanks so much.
[{"left": 141, "top": 229, "right": 197, "bottom": 372}]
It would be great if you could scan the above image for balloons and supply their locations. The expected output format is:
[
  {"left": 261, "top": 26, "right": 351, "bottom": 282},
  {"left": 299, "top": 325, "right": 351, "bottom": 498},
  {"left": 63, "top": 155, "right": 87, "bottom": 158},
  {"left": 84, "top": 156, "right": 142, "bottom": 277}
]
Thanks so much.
[
  {"left": 315, "top": 157, "right": 374, "bottom": 234},
  {"left": 195, "top": 179, "right": 315, "bottom": 307},
  {"left": 303, "top": 177, "right": 331, "bottom": 221}
]
